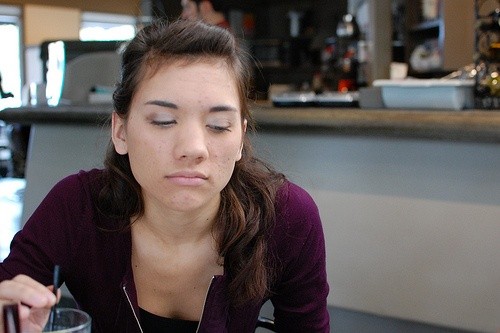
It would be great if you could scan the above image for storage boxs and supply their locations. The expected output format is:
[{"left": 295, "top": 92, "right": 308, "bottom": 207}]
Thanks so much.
[{"left": 372, "top": 79, "right": 473, "bottom": 111}]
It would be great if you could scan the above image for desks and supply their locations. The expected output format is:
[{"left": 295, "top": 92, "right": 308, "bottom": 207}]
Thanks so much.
[{"left": 1, "top": 103, "right": 500, "bottom": 333}]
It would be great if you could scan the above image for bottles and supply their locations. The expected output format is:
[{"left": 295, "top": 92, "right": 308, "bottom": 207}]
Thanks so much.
[
  {"left": 320, "top": 46, "right": 355, "bottom": 93},
  {"left": 472, "top": 64, "right": 500, "bottom": 110}
]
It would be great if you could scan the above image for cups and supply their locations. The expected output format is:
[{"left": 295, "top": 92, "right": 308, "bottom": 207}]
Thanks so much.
[{"left": 18, "top": 307, "right": 92, "bottom": 333}]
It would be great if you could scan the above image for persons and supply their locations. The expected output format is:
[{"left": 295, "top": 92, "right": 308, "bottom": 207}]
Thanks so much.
[{"left": 0, "top": 17, "right": 330, "bottom": 333}]
